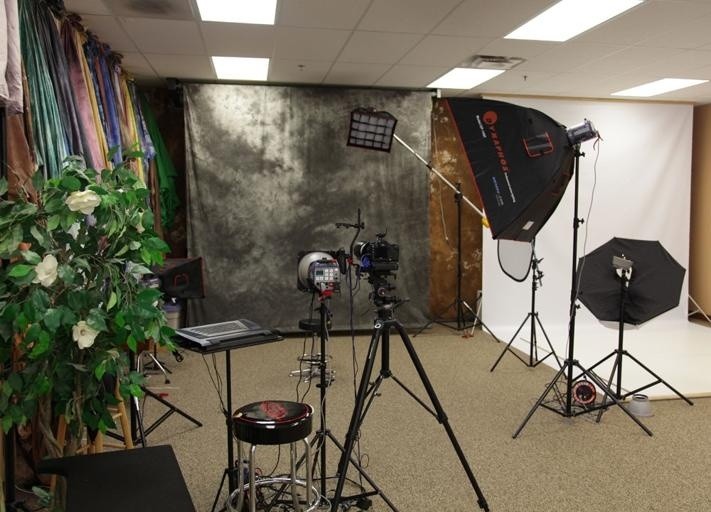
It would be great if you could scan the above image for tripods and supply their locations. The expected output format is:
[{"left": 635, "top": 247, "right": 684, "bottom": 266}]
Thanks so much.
[
  {"left": 491, "top": 257, "right": 567, "bottom": 380},
  {"left": 211, "top": 350, "right": 264, "bottom": 512},
  {"left": 412, "top": 179, "right": 500, "bottom": 344},
  {"left": 331, "top": 313, "right": 491, "bottom": 512},
  {"left": 88, "top": 373, "right": 202, "bottom": 447},
  {"left": 572, "top": 269, "right": 694, "bottom": 423},
  {"left": 512, "top": 150, "right": 654, "bottom": 439},
  {"left": 265, "top": 298, "right": 400, "bottom": 512}
]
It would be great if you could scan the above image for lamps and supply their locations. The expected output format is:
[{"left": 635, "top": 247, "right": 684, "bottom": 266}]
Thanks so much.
[{"left": 346, "top": 108, "right": 502, "bottom": 344}]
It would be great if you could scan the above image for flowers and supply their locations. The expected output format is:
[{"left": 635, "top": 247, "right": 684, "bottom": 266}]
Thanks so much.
[{"left": 1, "top": 139, "right": 178, "bottom": 458}]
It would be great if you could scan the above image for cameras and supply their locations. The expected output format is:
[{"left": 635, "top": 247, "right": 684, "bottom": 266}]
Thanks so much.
[{"left": 355, "top": 240, "right": 401, "bottom": 264}]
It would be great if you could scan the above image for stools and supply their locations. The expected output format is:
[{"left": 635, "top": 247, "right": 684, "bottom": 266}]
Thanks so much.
[{"left": 225, "top": 400, "right": 323, "bottom": 512}]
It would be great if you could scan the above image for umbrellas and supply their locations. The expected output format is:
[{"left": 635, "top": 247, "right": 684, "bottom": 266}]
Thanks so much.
[{"left": 575, "top": 236, "right": 687, "bottom": 327}]
